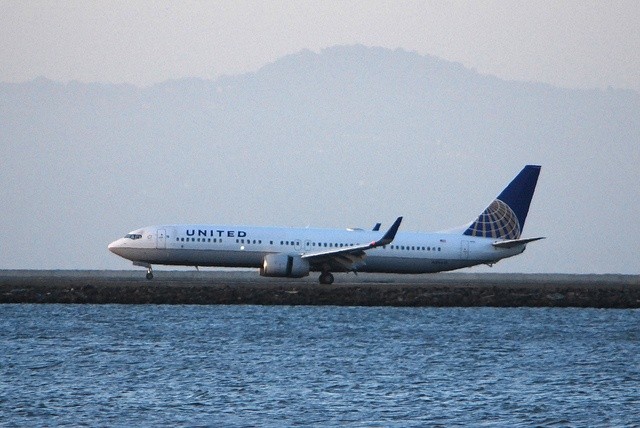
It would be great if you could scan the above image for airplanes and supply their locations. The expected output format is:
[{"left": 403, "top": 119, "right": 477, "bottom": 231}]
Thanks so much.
[{"left": 109, "top": 165, "right": 546, "bottom": 285}]
